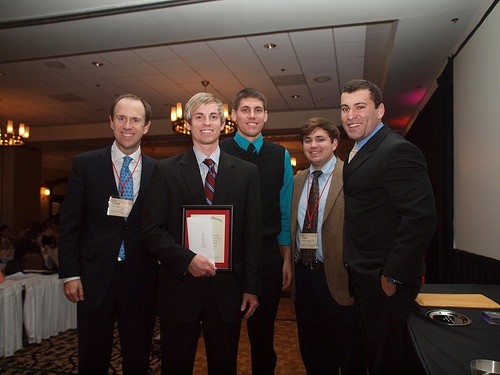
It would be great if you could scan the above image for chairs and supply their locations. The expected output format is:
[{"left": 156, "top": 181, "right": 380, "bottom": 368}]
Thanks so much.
[{"left": 20, "top": 250, "right": 43, "bottom": 271}]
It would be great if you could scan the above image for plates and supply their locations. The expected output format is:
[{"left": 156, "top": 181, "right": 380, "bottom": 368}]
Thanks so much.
[{"left": 426, "top": 309, "right": 472, "bottom": 326}]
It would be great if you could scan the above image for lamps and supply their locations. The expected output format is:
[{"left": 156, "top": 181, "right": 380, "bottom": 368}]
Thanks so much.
[
  {"left": 170, "top": 80, "right": 237, "bottom": 137},
  {"left": 0, "top": 119, "right": 31, "bottom": 147}
]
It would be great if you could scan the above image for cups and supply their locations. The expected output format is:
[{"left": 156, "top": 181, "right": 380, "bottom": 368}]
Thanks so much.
[{"left": 470, "top": 359, "right": 500, "bottom": 375}]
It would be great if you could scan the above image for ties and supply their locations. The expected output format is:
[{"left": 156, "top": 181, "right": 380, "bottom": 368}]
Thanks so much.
[
  {"left": 300, "top": 169, "right": 323, "bottom": 266},
  {"left": 117, "top": 156, "right": 135, "bottom": 261},
  {"left": 202, "top": 159, "right": 217, "bottom": 204},
  {"left": 246, "top": 143, "right": 257, "bottom": 160}
]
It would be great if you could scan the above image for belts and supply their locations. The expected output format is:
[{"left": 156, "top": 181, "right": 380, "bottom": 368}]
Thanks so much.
[{"left": 298, "top": 260, "right": 324, "bottom": 270}]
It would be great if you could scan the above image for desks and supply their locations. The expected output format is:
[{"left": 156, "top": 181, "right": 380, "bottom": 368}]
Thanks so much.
[
  {"left": 408, "top": 285, "right": 500, "bottom": 375},
  {"left": 0, "top": 268, "right": 78, "bottom": 359}
]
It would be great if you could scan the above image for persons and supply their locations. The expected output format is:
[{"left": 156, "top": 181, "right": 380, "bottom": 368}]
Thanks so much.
[
  {"left": 219, "top": 88, "right": 294, "bottom": 375},
  {"left": 290, "top": 118, "right": 367, "bottom": 375},
  {"left": 58, "top": 93, "right": 159, "bottom": 375},
  {"left": 340, "top": 79, "right": 437, "bottom": 375},
  {"left": 141, "top": 92, "right": 263, "bottom": 375},
  {"left": 0, "top": 223, "right": 60, "bottom": 277}
]
